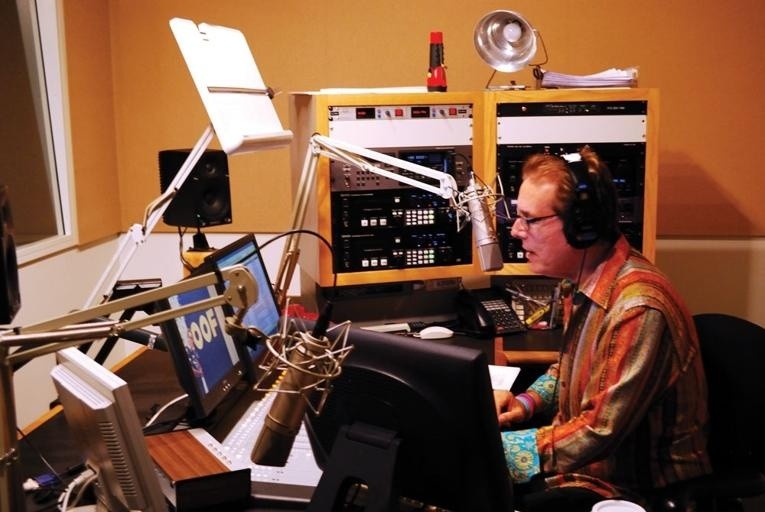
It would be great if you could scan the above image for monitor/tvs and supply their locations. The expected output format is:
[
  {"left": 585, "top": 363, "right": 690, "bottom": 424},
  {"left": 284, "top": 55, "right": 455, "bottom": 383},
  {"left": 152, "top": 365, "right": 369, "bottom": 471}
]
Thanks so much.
[
  {"left": 159, "top": 262, "right": 245, "bottom": 420},
  {"left": 197, "top": 232, "right": 283, "bottom": 362},
  {"left": 277, "top": 318, "right": 515, "bottom": 512},
  {"left": 51, "top": 345, "right": 169, "bottom": 512}
]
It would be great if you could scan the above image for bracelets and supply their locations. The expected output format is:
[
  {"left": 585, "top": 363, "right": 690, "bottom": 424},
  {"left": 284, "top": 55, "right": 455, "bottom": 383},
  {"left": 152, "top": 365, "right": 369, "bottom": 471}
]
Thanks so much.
[{"left": 514, "top": 393, "right": 537, "bottom": 421}]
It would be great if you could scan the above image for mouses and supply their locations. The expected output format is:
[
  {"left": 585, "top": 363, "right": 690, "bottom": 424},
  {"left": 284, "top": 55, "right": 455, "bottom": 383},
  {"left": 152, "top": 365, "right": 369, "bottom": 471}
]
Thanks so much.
[{"left": 419, "top": 325, "right": 453, "bottom": 339}]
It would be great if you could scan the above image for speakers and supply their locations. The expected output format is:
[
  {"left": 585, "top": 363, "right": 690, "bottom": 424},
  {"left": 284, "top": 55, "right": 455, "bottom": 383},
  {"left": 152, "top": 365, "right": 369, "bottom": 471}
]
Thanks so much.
[
  {"left": 0, "top": 185, "right": 22, "bottom": 324},
  {"left": 160, "top": 149, "right": 231, "bottom": 227}
]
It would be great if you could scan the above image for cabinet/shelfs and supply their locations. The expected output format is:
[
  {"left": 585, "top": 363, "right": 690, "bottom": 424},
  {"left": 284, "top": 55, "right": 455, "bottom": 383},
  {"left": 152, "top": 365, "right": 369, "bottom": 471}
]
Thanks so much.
[{"left": 282, "top": 84, "right": 663, "bottom": 325}]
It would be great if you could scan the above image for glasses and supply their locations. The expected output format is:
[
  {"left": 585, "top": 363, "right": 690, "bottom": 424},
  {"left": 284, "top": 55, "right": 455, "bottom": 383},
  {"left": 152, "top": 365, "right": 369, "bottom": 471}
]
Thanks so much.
[{"left": 515, "top": 208, "right": 567, "bottom": 232}]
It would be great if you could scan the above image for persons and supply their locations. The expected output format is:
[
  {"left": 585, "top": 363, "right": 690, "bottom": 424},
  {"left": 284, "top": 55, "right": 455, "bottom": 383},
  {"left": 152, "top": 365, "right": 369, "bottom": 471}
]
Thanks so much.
[{"left": 492, "top": 145, "right": 711, "bottom": 511}]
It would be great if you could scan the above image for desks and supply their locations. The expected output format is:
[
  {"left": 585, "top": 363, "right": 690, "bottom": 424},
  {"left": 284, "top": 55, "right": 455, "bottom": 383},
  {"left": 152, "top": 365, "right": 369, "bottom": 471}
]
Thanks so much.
[{"left": 2, "top": 310, "right": 579, "bottom": 510}]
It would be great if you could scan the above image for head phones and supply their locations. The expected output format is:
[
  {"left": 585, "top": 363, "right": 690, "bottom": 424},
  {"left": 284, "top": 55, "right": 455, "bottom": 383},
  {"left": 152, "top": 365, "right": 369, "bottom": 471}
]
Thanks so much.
[{"left": 557, "top": 152, "right": 614, "bottom": 250}]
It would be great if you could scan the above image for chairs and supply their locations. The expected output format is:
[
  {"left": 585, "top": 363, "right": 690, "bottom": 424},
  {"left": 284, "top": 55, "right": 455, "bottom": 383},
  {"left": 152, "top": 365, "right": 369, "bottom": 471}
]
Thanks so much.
[{"left": 690, "top": 312, "right": 765, "bottom": 510}]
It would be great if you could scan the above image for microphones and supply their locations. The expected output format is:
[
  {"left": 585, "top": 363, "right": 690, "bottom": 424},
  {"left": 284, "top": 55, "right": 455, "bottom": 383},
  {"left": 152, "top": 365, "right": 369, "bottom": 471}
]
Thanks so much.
[
  {"left": 464, "top": 182, "right": 504, "bottom": 273},
  {"left": 250, "top": 330, "right": 330, "bottom": 466}
]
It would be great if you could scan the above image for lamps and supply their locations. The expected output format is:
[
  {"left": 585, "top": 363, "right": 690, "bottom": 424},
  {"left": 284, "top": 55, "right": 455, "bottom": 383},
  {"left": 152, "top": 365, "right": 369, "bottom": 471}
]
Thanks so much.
[{"left": 471, "top": 8, "right": 553, "bottom": 92}]
